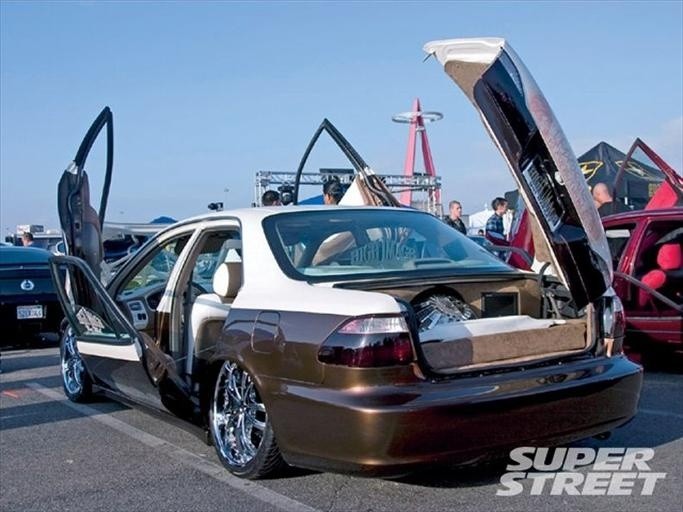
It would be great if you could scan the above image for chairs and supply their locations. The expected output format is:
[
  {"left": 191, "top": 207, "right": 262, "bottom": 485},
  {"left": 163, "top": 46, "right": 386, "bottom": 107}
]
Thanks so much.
[{"left": 181, "top": 261, "right": 244, "bottom": 373}]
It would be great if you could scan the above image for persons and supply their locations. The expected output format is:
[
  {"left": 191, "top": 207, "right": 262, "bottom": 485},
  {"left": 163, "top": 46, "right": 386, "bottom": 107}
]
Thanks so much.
[
  {"left": 592, "top": 182, "right": 637, "bottom": 271},
  {"left": 21, "top": 232, "right": 34, "bottom": 247},
  {"left": 485, "top": 198, "right": 509, "bottom": 261},
  {"left": 322, "top": 179, "right": 344, "bottom": 205},
  {"left": 441, "top": 201, "right": 467, "bottom": 235},
  {"left": 262, "top": 190, "right": 281, "bottom": 206}
]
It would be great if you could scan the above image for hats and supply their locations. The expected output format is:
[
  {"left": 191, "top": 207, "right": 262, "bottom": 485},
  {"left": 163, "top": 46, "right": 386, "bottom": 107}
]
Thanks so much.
[{"left": 324, "top": 179, "right": 347, "bottom": 196}]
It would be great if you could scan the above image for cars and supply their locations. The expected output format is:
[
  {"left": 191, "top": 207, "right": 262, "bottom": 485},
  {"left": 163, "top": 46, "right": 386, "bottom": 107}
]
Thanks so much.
[{"left": 0, "top": 39, "right": 683, "bottom": 483}]
